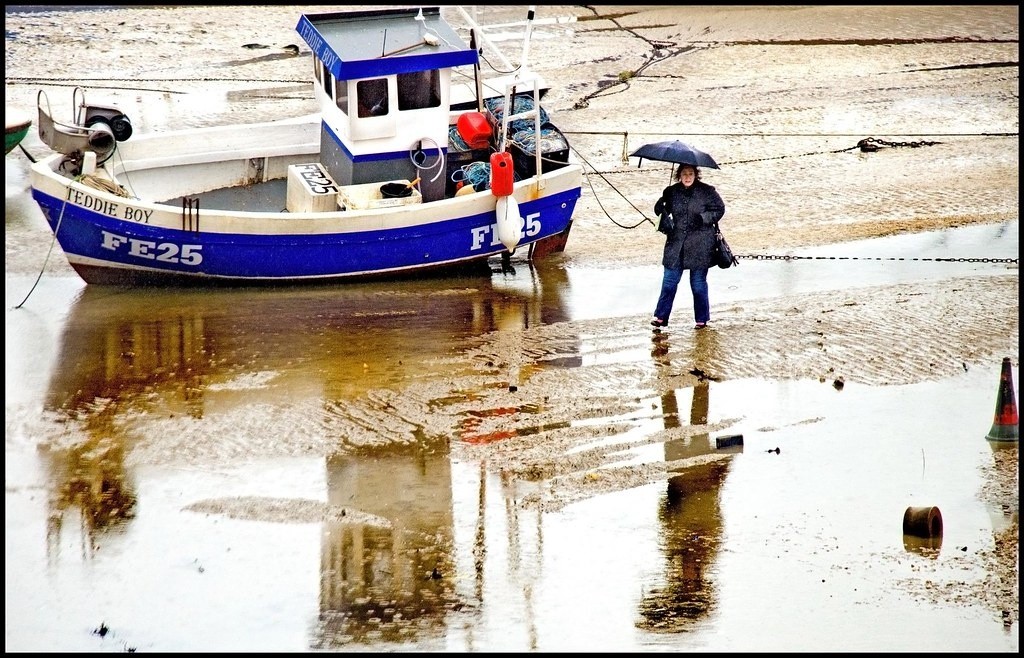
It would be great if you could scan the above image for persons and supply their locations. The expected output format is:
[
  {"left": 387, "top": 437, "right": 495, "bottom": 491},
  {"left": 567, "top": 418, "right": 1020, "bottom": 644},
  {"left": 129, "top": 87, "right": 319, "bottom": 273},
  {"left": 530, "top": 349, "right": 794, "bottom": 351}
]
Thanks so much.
[
  {"left": 651, "top": 163, "right": 725, "bottom": 329},
  {"left": 650, "top": 329, "right": 732, "bottom": 582}
]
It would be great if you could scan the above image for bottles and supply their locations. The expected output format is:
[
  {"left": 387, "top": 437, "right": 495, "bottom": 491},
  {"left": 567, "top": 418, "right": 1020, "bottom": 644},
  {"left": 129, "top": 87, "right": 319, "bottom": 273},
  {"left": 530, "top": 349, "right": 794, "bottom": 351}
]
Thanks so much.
[
  {"left": 489, "top": 152, "right": 515, "bottom": 196},
  {"left": 457, "top": 113, "right": 493, "bottom": 149}
]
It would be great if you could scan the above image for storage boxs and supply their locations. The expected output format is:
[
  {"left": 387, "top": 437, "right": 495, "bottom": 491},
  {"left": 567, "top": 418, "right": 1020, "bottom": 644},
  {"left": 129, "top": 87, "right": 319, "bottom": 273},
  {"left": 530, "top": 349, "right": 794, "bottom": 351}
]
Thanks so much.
[
  {"left": 485, "top": 93, "right": 549, "bottom": 150},
  {"left": 509, "top": 122, "right": 569, "bottom": 178},
  {"left": 337, "top": 179, "right": 422, "bottom": 211}
]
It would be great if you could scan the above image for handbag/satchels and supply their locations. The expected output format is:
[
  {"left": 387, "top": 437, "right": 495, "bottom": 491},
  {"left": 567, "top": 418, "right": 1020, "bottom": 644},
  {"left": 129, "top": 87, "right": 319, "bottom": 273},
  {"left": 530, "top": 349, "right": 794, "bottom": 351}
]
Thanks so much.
[
  {"left": 712, "top": 221, "right": 739, "bottom": 269},
  {"left": 657, "top": 208, "right": 673, "bottom": 233}
]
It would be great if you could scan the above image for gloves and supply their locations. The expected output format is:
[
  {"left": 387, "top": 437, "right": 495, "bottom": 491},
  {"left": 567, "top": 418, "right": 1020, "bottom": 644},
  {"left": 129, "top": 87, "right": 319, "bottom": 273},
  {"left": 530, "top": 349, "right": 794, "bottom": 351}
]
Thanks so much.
[{"left": 693, "top": 213, "right": 704, "bottom": 230}]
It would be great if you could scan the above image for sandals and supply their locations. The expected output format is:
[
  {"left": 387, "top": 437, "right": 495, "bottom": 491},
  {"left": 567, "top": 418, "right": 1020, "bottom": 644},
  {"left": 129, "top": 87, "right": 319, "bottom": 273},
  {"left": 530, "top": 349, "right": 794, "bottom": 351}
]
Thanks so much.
[
  {"left": 651, "top": 318, "right": 667, "bottom": 327},
  {"left": 695, "top": 322, "right": 705, "bottom": 329}
]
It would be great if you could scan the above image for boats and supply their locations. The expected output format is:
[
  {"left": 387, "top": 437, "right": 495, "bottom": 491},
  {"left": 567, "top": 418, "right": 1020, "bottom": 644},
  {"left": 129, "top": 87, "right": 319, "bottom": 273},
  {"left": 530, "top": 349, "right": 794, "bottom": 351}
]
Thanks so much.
[{"left": 25, "top": 7, "right": 583, "bottom": 285}]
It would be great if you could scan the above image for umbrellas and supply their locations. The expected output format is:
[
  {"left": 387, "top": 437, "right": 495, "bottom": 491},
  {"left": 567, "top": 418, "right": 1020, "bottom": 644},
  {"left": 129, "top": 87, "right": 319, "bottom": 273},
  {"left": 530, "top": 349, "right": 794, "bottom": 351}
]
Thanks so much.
[
  {"left": 635, "top": 584, "right": 716, "bottom": 634},
  {"left": 628, "top": 139, "right": 722, "bottom": 189}
]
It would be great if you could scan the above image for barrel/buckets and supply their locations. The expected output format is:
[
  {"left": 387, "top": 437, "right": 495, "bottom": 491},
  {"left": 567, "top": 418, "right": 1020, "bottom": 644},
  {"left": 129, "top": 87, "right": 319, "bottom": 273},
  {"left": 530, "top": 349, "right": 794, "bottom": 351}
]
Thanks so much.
[{"left": 380, "top": 183, "right": 413, "bottom": 198}]
